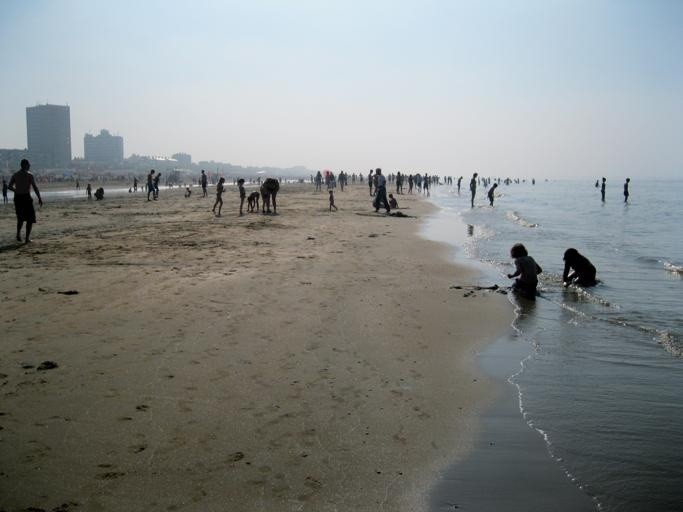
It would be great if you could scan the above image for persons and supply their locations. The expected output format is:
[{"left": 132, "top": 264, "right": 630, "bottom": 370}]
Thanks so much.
[
  {"left": 562, "top": 248, "right": 596, "bottom": 286},
  {"left": 328, "top": 190, "right": 337, "bottom": 211},
  {"left": 623, "top": 178, "right": 630, "bottom": 202},
  {"left": 201, "top": 170, "right": 207, "bottom": 198},
  {"left": 87, "top": 185, "right": 104, "bottom": 201},
  {"left": 498, "top": 177, "right": 535, "bottom": 185},
  {"left": 595, "top": 179, "right": 599, "bottom": 187},
  {"left": 315, "top": 167, "right": 496, "bottom": 207},
  {"left": 238, "top": 178, "right": 280, "bottom": 213},
  {"left": 601, "top": 178, "right": 606, "bottom": 202},
  {"left": 9, "top": 159, "right": 43, "bottom": 243},
  {"left": 134, "top": 169, "right": 161, "bottom": 201},
  {"left": 212, "top": 177, "right": 226, "bottom": 213},
  {"left": 187, "top": 187, "right": 191, "bottom": 197},
  {"left": 507, "top": 242, "right": 542, "bottom": 301},
  {"left": 375, "top": 168, "right": 390, "bottom": 212}
]
